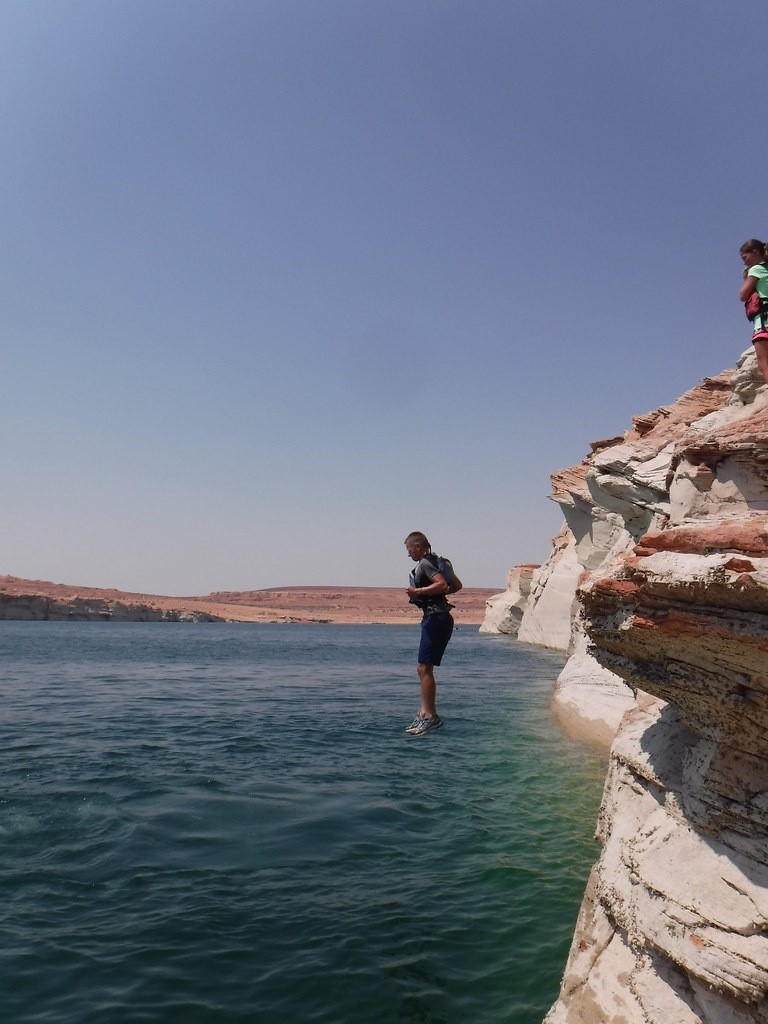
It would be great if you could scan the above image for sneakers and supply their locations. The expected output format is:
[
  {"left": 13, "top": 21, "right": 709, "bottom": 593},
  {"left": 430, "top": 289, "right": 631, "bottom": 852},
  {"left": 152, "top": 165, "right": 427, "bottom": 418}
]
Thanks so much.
[
  {"left": 405, "top": 711, "right": 424, "bottom": 733},
  {"left": 411, "top": 716, "right": 443, "bottom": 736}
]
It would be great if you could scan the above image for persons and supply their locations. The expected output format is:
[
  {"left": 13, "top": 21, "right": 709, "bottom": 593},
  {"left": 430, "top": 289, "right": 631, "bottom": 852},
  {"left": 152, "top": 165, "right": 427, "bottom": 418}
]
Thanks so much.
[
  {"left": 404, "top": 532, "right": 462, "bottom": 735},
  {"left": 739, "top": 239, "right": 768, "bottom": 384}
]
